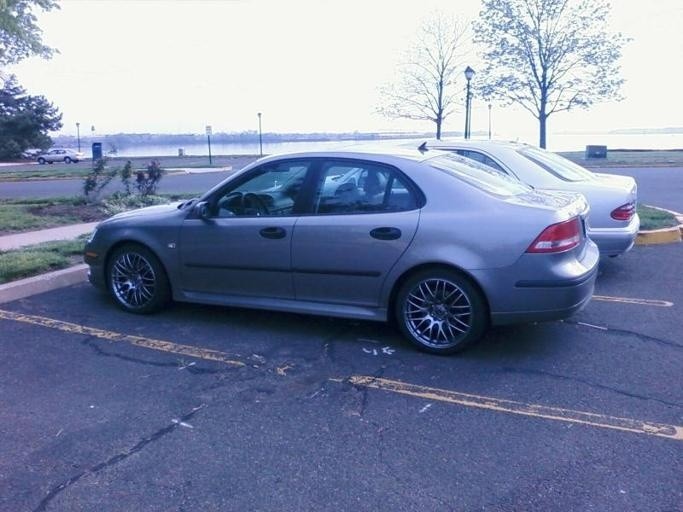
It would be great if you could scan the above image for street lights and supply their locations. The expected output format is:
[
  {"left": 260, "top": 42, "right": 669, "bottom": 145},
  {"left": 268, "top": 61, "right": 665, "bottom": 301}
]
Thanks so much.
[
  {"left": 257, "top": 113, "right": 263, "bottom": 158},
  {"left": 76, "top": 122, "right": 81, "bottom": 152},
  {"left": 488, "top": 104, "right": 492, "bottom": 140},
  {"left": 464, "top": 66, "right": 475, "bottom": 139}
]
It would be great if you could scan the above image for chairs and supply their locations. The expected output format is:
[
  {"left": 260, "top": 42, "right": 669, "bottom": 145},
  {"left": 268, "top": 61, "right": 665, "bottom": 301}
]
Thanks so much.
[{"left": 334, "top": 169, "right": 387, "bottom": 206}]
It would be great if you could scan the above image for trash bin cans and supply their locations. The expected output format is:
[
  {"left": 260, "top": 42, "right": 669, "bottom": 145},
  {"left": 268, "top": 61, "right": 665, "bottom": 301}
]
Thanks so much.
[
  {"left": 179, "top": 148, "right": 183, "bottom": 156},
  {"left": 92, "top": 142, "right": 101, "bottom": 160},
  {"left": 585, "top": 145, "right": 607, "bottom": 158}
]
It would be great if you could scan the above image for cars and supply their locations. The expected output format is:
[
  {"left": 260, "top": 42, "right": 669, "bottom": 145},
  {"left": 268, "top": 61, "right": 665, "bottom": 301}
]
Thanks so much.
[{"left": 22, "top": 149, "right": 84, "bottom": 163}]
